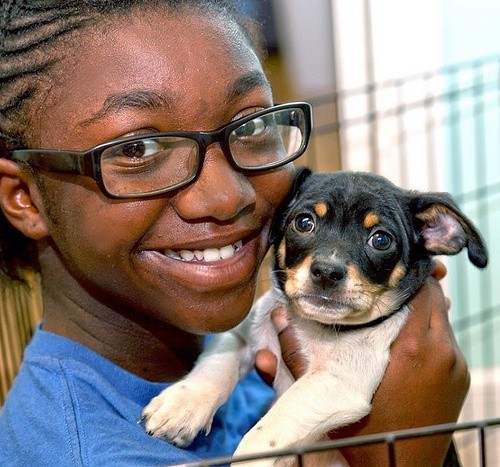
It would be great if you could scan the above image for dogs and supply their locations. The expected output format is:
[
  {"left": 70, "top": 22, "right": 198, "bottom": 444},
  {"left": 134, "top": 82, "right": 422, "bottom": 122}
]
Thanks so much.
[{"left": 135, "top": 166, "right": 489, "bottom": 466}]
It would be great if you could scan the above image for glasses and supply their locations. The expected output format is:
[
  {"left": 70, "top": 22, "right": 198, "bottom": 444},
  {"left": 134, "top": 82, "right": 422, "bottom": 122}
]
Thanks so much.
[{"left": 6, "top": 102, "right": 312, "bottom": 199}]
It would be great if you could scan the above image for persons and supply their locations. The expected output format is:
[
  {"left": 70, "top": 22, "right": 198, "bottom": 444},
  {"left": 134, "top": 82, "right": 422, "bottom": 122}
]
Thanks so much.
[{"left": 0, "top": 0, "right": 470, "bottom": 467}]
